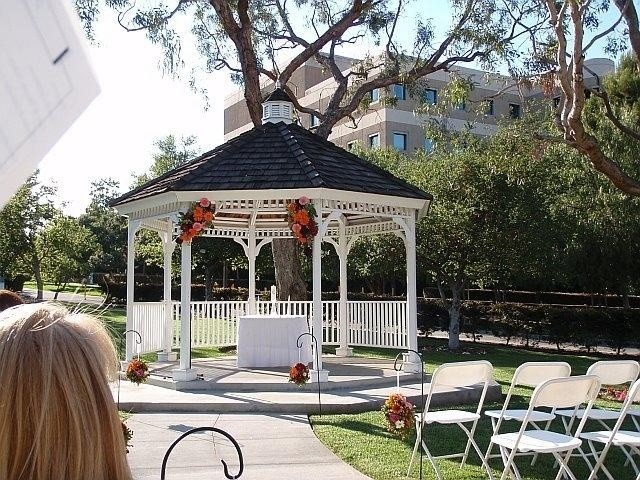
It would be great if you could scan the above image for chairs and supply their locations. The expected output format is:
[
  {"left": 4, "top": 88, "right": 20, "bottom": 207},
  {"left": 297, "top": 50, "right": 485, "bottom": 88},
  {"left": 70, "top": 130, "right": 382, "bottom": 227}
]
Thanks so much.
[{"left": 405, "top": 357, "right": 639, "bottom": 479}]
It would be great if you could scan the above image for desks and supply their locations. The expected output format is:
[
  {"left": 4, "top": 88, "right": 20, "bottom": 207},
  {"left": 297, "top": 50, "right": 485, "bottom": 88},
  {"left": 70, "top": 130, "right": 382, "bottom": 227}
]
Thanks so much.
[{"left": 236, "top": 314, "right": 313, "bottom": 369}]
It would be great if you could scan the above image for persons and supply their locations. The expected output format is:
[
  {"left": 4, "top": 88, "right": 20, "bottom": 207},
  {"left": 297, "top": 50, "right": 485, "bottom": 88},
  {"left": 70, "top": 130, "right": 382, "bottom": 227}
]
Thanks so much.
[
  {"left": 0, "top": 289, "right": 25, "bottom": 312},
  {"left": 0, "top": 302, "right": 135, "bottom": 480}
]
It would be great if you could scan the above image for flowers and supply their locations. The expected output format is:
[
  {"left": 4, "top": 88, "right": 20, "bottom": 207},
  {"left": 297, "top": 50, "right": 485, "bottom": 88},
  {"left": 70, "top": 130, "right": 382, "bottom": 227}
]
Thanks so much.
[
  {"left": 381, "top": 393, "right": 417, "bottom": 440},
  {"left": 124, "top": 355, "right": 152, "bottom": 387},
  {"left": 174, "top": 197, "right": 215, "bottom": 246},
  {"left": 287, "top": 361, "right": 311, "bottom": 387},
  {"left": 284, "top": 196, "right": 320, "bottom": 256}
]
